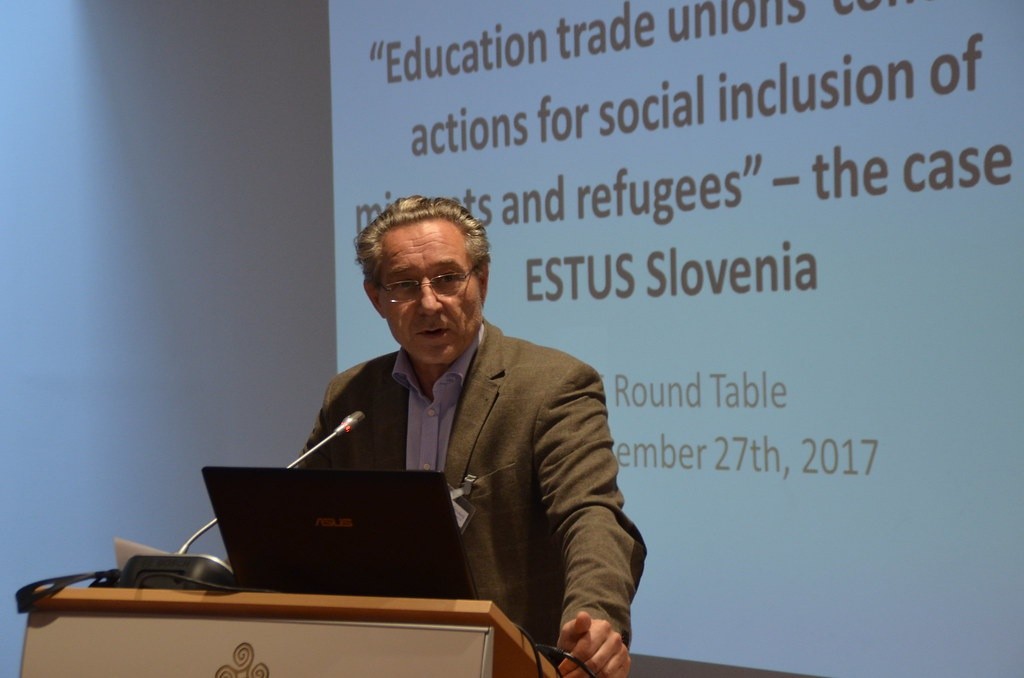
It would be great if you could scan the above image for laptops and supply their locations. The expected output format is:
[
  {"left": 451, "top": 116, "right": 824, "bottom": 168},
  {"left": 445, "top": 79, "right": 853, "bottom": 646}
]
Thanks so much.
[{"left": 201, "top": 465, "right": 477, "bottom": 604}]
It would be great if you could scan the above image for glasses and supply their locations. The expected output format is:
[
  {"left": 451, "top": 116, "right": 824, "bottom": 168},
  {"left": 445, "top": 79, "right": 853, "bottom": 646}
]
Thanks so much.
[{"left": 377, "top": 260, "right": 481, "bottom": 303}]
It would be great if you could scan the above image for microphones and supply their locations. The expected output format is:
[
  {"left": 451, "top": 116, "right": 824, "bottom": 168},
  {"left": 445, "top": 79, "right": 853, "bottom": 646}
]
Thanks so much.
[{"left": 121, "top": 410, "right": 366, "bottom": 590}]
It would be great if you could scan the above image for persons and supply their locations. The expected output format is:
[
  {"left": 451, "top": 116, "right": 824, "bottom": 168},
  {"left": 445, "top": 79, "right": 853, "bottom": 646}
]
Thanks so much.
[{"left": 291, "top": 195, "right": 647, "bottom": 678}]
